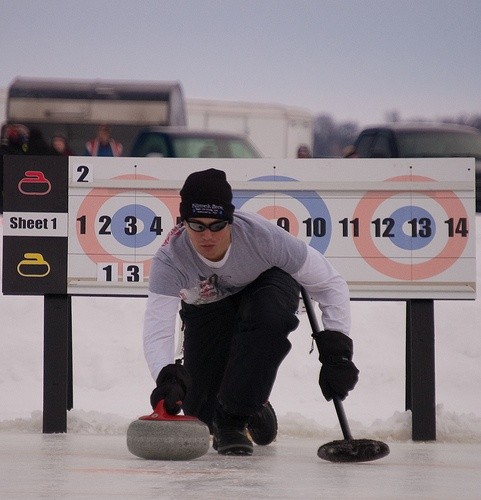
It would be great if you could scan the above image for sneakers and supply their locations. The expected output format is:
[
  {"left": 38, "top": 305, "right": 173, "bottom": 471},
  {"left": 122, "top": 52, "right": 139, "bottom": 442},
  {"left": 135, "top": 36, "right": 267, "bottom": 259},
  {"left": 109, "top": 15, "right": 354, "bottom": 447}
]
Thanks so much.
[
  {"left": 247, "top": 400, "right": 278, "bottom": 446},
  {"left": 212, "top": 422, "right": 254, "bottom": 457}
]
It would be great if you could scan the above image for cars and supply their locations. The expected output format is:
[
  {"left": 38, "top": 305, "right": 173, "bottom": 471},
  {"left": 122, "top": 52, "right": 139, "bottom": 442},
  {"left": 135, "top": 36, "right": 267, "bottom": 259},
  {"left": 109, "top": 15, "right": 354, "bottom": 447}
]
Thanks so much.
[
  {"left": 119, "top": 128, "right": 264, "bottom": 160},
  {"left": 346, "top": 124, "right": 481, "bottom": 213}
]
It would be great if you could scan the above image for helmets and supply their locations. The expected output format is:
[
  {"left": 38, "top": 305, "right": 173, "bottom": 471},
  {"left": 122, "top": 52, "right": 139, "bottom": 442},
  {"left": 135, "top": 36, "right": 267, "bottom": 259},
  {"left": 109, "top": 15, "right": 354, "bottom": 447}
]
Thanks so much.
[{"left": 3, "top": 124, "right": 29, "bottom": 154}]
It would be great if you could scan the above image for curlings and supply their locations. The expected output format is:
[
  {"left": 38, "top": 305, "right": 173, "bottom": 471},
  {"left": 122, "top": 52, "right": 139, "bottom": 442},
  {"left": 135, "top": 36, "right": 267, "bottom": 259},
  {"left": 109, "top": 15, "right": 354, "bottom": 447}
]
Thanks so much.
[{"left": 127, "top": 399, "right": 210, "bottom": 461}]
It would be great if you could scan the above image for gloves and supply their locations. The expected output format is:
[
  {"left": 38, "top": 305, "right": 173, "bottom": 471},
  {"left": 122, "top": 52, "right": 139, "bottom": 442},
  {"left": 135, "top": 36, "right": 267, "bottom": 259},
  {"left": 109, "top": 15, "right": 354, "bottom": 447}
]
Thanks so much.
[
  {"left": 149, "top": 378, "right": 198, "bottom": 415},
  {"left": 314, "top": 330, "right": 359, "bottom": 402}
]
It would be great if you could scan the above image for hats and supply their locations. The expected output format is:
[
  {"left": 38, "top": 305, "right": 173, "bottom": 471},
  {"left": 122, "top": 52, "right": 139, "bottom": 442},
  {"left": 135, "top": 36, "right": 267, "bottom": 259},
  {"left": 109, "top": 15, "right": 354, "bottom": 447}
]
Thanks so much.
[{"left": 179, "top": 168, "right": 235, "bottom": 224}]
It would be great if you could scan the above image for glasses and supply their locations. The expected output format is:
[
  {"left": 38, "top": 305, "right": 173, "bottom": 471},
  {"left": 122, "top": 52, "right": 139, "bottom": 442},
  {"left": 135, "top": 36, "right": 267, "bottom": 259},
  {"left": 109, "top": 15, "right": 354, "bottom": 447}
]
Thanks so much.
[{"left": 185, "top": 218, "right": 230, "bottom": 232}]
